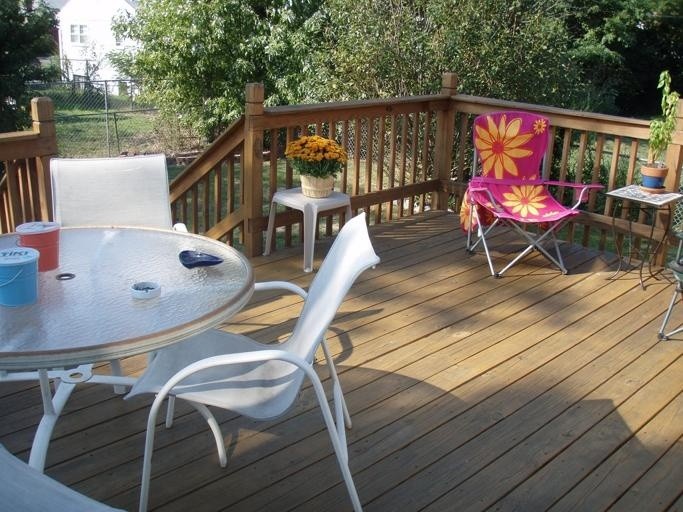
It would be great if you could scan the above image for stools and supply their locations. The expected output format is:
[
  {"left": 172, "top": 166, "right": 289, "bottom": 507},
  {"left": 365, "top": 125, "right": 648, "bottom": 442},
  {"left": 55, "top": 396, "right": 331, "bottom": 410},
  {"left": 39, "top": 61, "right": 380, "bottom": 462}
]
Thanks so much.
[{"left": 261, "top": 184, "right": 352, "bottom": 273}]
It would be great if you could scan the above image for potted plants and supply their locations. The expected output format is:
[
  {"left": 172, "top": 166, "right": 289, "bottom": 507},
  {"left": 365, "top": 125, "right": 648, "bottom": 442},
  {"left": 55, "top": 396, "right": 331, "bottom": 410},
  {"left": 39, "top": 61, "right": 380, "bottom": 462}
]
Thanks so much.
[{"left": 637, "top": 68, "right": 681, "bottom": 195}]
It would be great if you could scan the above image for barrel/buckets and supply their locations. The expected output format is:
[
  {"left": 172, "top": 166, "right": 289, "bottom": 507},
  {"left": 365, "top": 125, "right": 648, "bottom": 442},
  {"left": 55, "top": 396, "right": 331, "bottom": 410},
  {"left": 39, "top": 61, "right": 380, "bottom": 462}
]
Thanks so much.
[
  {"left": 15, "top": 221, "right": 61, "bottom": 273},
  {"left": 0, "top": 247, "right": 41, "bottom": 307}
]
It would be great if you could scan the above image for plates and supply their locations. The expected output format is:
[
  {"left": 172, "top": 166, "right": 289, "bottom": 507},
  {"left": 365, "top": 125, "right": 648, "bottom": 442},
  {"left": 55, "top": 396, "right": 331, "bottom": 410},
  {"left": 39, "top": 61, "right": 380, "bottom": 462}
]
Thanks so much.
[{"left": 179, "top": 249, "right": 224, "bottom": 270}]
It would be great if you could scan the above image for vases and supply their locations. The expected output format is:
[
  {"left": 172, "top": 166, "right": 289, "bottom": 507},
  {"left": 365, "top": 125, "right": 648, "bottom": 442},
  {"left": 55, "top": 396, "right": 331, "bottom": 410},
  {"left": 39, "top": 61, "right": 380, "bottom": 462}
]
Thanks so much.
[{"left": 298, "top": 169, "right": 336, "bottom": 199}]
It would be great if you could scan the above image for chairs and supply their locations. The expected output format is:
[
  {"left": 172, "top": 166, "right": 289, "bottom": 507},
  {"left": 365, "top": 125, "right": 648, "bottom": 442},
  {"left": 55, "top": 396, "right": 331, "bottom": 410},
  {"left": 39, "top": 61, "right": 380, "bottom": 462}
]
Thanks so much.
[
  {"left": 0, "top": 438, "right": 127, "bottom": 511},
  {"left": 120, "top": 212, "right": 379, "bottom": 512},
  {"left": 47, "top": 154, "right": 180, "bottom": 429},
  {"left": 658, "top": 228, "right": 681, "bottom": 342},
  {"left": 455, "top": 111, "right": 604, "bottom": 278}
]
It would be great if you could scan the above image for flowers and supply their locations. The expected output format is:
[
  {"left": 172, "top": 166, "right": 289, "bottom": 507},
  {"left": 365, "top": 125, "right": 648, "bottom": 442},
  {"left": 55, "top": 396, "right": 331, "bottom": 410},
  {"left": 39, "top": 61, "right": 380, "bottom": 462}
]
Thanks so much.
[{"left": 281, "top": 133, "right": 349, "bottom": 180}]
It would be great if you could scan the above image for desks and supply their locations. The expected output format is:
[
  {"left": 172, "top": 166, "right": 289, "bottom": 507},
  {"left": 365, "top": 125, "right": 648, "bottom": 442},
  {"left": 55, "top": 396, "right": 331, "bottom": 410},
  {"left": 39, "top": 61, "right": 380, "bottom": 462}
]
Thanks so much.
[
  {"left": 0, "top": 222, "right": 256, "bottom": 477},
  {"left": 601, "top": 182, "right": 681, "bottom": 289}
]
